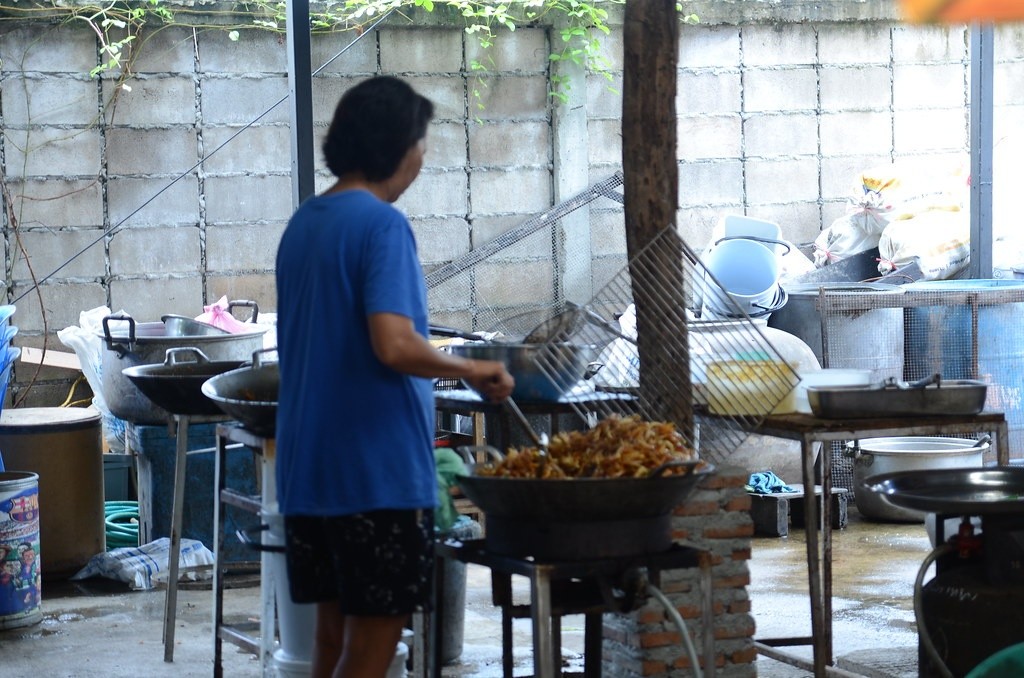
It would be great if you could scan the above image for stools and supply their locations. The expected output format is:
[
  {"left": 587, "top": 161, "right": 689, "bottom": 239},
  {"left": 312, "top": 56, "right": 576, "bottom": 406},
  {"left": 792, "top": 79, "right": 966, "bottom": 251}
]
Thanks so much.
[
  {"left": 161, "top": 411, "right": 240, "bottom": 661},
  {"left": 750, "top": 483, "right": 848, "bottom": 539}
]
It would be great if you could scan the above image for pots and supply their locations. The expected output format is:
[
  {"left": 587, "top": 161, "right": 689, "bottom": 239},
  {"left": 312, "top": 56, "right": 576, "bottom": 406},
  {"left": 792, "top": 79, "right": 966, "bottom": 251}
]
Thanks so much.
[
  {"left": 94, "top": 300, "right": 278, "bottom": 439},
  {"left": 453, "top": 445, "right": 716, "bottom": 542},
  {"left": 841, "top": 434, "right": 992, "bottom": 525},
  {"left": 441, "top": 339, "right": 598, "bottom": 402}
]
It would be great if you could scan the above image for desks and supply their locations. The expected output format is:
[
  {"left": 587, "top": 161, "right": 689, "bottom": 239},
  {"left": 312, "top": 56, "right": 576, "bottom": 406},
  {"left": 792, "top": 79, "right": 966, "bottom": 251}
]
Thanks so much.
[
  {"left": 693, "top": 403, "right": 1009, "bottom": 678},
  {"left": 435, "top": 389, "right": 637, "bottom": 607}
]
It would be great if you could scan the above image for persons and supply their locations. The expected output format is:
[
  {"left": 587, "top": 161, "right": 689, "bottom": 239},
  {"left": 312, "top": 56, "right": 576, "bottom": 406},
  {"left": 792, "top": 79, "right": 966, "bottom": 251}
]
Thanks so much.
[{"left": 275, "top": 74, "right": 515, "bottom": 678}]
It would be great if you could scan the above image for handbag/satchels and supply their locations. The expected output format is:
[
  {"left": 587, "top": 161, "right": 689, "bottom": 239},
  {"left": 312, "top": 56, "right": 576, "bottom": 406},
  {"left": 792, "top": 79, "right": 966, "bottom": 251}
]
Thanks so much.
[{"left": 72, "top": 536, "right": 215, "bottom": 595}]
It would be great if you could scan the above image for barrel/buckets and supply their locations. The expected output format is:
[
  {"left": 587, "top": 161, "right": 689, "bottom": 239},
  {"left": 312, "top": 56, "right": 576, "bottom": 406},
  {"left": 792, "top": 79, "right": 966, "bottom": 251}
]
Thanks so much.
[
  {"left": 904, "top": 278, "right": 1024, "bottom": 460},
  {"left": 691, "top": 214, "right": 791, "bottom": 324},
  {"left": 0, "top": 470, "right": 44, "bottom": 628},
  {"left": 771, "top": 280, "right": 903, "bottom": 382}
]
